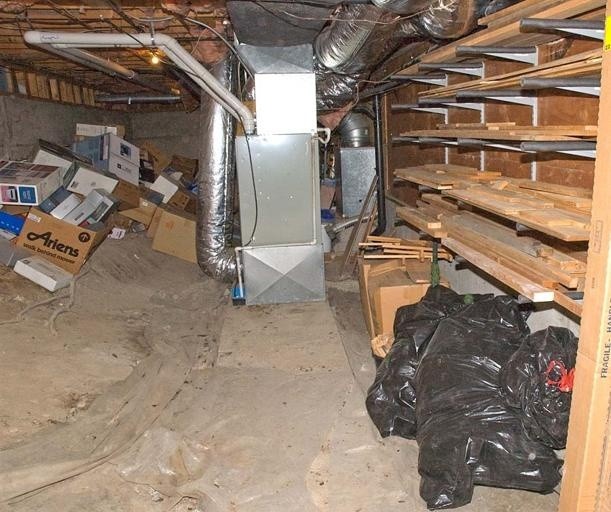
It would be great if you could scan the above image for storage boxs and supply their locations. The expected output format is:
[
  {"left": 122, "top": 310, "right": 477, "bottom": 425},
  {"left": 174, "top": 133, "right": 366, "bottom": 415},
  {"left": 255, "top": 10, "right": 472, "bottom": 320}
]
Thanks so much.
[
  {"left": 0, "top": 121, "right": 203, "bottom": 291},
  {"left": 361, "top": 259, "right": 449, "bottom": 345}
]
